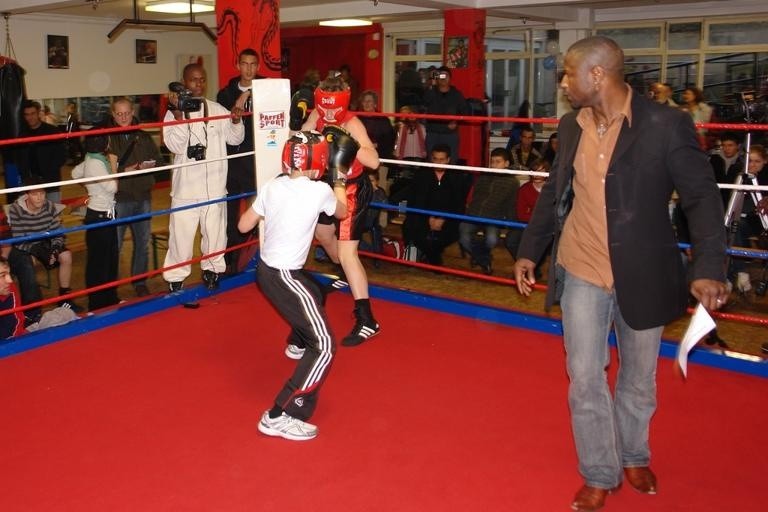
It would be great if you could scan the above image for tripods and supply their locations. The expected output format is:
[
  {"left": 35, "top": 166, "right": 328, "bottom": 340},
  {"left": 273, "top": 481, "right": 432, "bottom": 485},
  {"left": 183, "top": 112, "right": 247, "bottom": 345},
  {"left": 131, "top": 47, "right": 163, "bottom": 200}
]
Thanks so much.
[{"left": 719, "top": 130, "right": 768, "bottom": 240}]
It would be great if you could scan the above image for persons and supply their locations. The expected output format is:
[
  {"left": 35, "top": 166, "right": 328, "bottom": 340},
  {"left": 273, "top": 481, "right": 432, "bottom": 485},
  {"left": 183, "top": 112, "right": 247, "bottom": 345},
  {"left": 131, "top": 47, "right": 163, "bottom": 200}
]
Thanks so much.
[
  {"left": 294, "top": 65, "right": 559, "bottom": 281},
  {"left": 512, "top": 35, "right": 730, "bottom": 512},
  {"left": 237, "top": 130, "right": 361, "bottom": 439},
  {"left": 0, "top": 50, "right": 268, "bottom": 338},
  {"left": 646, "top": 81, "right": 768, "bottom": 301}
]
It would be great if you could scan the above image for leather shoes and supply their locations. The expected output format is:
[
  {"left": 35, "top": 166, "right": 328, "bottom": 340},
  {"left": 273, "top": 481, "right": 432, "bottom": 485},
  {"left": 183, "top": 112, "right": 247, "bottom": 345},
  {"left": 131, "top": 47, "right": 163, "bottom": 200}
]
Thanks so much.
[
  {"left": 571, "top": 482, "right": 623, "bottom": 511},
  {"left": 623, "top": 467, "right": 657, "bottom": 495}
]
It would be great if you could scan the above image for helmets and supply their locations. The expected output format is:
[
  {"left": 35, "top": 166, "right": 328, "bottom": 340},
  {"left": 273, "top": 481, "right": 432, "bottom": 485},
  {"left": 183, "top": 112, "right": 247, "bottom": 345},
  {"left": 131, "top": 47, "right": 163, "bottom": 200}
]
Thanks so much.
[
  {"left": 281, "top": 130, "right": 330, "bottom": 180},
  {"left": 314, "top": 77, "right": 353, "bottom": 125}
]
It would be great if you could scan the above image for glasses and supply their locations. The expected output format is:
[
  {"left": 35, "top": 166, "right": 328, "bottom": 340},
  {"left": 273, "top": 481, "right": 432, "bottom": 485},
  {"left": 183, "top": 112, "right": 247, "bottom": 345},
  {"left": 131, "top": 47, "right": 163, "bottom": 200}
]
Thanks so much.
[{"left": 115, "top": 111, "right": 132, "bottom": 116}]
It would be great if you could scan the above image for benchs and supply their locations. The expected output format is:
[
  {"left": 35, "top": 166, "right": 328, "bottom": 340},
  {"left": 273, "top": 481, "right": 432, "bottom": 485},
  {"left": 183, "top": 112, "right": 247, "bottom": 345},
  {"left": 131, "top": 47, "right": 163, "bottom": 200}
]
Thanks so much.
[{"left": 0, "top": 180, "right": 172, "bottom": 289}]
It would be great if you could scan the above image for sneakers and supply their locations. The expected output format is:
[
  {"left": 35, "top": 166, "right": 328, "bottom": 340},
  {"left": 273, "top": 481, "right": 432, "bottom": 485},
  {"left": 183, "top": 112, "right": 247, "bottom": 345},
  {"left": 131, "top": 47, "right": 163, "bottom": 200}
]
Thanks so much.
[
  {"left": 24, "top": 315, "right": 41, "bottom": 327},
  {"left": 324, "top": 278, "right": 349, "bottom": 292},
  {"left": 257, "top": 408, "right": 319, "bottom": 441},
  {"left": 136, "top": 286, "right": 150, "bottom": 297},
  {"left": 341, "top": 319, "right": 382, "bottom": 347},
  {"left": 57, "top": 300, "right": 84, "bottom": 313},
  {"left": 285, "top": 343, "right": 307, "bottom": 360},
  {"left": 202, "top": 270, "right": 219, "bottom": 290},
  {"left": 169, "top": 282, "right": 199, "bottom": 308}
]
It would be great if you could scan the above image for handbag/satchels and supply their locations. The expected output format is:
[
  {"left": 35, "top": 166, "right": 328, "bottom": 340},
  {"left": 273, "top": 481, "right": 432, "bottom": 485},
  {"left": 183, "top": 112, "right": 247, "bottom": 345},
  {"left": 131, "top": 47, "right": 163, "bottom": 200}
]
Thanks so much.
[
  {"left": 402, "top": 242, "right": 422, "bottom": 263},
  {"left": 381, "top": 235, "right": 405, "bottom": 259}
]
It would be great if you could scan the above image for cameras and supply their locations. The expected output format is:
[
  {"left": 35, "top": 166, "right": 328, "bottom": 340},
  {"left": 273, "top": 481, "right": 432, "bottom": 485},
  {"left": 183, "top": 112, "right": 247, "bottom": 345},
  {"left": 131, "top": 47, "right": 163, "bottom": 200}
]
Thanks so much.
[
  {"left": 167, "top": 82, "right": 202, "bottom": 113},
  {"left": 432, "top": 73, "right": 448, "bottom": 81},
  {"left": 734, "top": 86, "right": 768, "bottom": 127},
  {"left": 186, "top": 144, "right": 207, "bottom": 165}
]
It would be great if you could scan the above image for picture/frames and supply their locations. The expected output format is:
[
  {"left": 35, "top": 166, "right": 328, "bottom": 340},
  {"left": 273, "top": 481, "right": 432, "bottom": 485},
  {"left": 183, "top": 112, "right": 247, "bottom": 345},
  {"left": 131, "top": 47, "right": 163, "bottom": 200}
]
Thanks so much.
[
  {"left": 136, "top": 38, "right": 158, "bottom": 64},
  {"left": 47, "top": 34, "right": 70, "bottom": 69}
]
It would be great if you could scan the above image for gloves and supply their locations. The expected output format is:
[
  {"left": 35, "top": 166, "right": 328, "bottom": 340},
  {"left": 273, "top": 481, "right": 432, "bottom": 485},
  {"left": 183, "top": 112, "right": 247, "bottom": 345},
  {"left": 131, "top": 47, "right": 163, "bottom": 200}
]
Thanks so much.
[
  {"left": 290, "top": 87, "right": 313, "bottom": 132},
  {"left": 323, "top": 125, "right": 361, "bottom": 187}
]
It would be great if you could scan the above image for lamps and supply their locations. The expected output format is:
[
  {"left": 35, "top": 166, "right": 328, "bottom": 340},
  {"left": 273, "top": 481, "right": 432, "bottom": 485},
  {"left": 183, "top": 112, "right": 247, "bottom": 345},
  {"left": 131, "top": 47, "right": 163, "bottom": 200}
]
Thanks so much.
[
  {"left": 145, "top": 0, "right": 215, "bottom": 14},
  {"left": 319, "top": 18, "right": 373, "bottom": 27}
]
